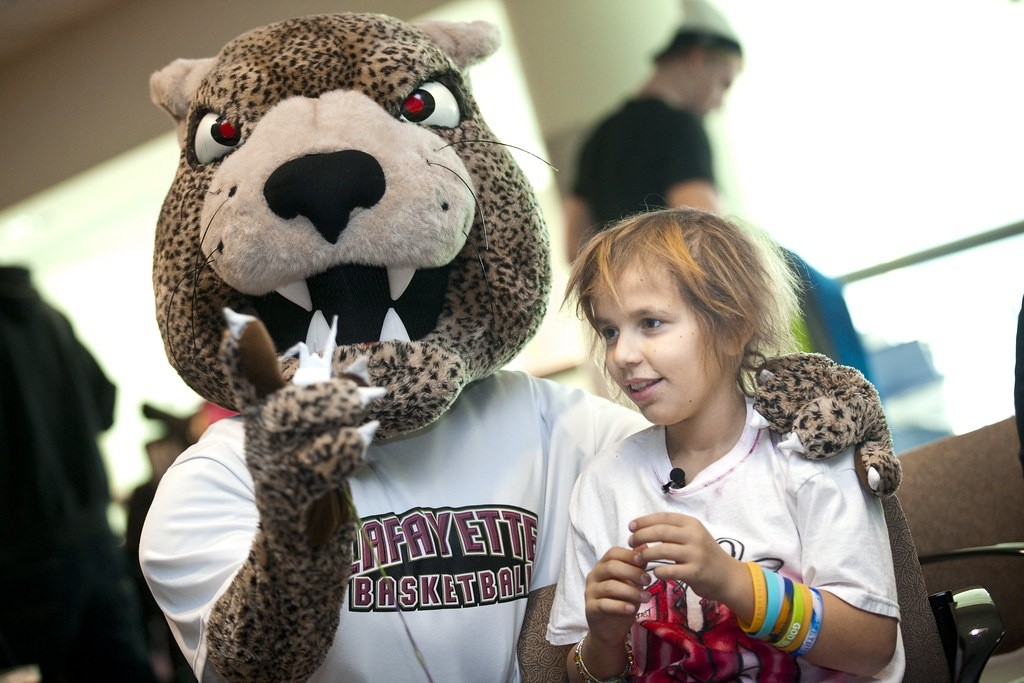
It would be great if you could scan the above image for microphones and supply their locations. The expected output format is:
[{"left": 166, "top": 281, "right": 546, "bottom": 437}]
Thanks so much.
[{"left": 670, "top": 468, "right": 685, "bottom": 488}]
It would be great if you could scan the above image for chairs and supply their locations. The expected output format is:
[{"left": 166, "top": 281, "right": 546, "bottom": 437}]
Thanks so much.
[{"left": 882, "top": 496, "right": 1006, "bottom": 683}]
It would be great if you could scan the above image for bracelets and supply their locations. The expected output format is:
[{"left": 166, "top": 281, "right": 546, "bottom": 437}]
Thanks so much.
[
  {"left": 737, "top": 561, "right": 823, "bottom": 656},
  {"left": 574, "top": 636, "right": 635, "bottom": 683}
]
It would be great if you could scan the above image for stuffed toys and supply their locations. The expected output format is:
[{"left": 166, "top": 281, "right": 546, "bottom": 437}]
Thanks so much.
[{"left": 137, "top": 11, "right": 902, "bottom": 683}]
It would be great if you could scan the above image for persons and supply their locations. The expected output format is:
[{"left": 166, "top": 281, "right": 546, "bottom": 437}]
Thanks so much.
[
  {"left": 0, "top": 265, "right": 157, "bottom": 683},
  {"left": 544, "top": 209, "right": 906, "bottom": 683},
  {"left": 562, "top": 29, "right": 744, "bottom": 268}
]
